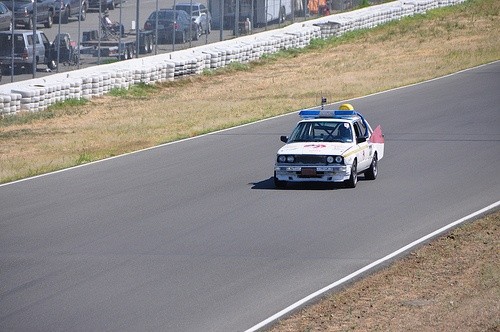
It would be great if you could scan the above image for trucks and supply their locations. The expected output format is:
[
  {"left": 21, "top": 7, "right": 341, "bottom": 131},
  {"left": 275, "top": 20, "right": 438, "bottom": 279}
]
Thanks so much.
[{"left": 208, "top": 0, "right": 310, "bottom": 30}]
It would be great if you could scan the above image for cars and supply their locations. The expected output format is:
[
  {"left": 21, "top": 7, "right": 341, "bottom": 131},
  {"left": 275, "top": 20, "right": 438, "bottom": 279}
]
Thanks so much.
[
  {"left": 144, "top": 8, "right": 200, "bottom": 44},
  {"left": 6, "top": 0, "right": 126, "bottom": 30},
  {"left": 273, "top": 97, "right": 385, "bottom": 190},
  {"left": 0, "top": 1, "right": 13, "bottom": 31},
  {"left": 171, "top": 3, "right": 211, "bottom": 38}
]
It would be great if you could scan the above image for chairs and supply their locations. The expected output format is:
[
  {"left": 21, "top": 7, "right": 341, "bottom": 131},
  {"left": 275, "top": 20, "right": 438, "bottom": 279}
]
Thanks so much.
[{"left": 338, "top": 125, "right": 350, "bottom": 136}]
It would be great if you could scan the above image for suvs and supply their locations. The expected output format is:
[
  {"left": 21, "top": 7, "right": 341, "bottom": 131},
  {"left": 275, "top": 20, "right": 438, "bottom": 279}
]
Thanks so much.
[{"left": 0, "top": 29, "right": 73, "bottom": 76}]
